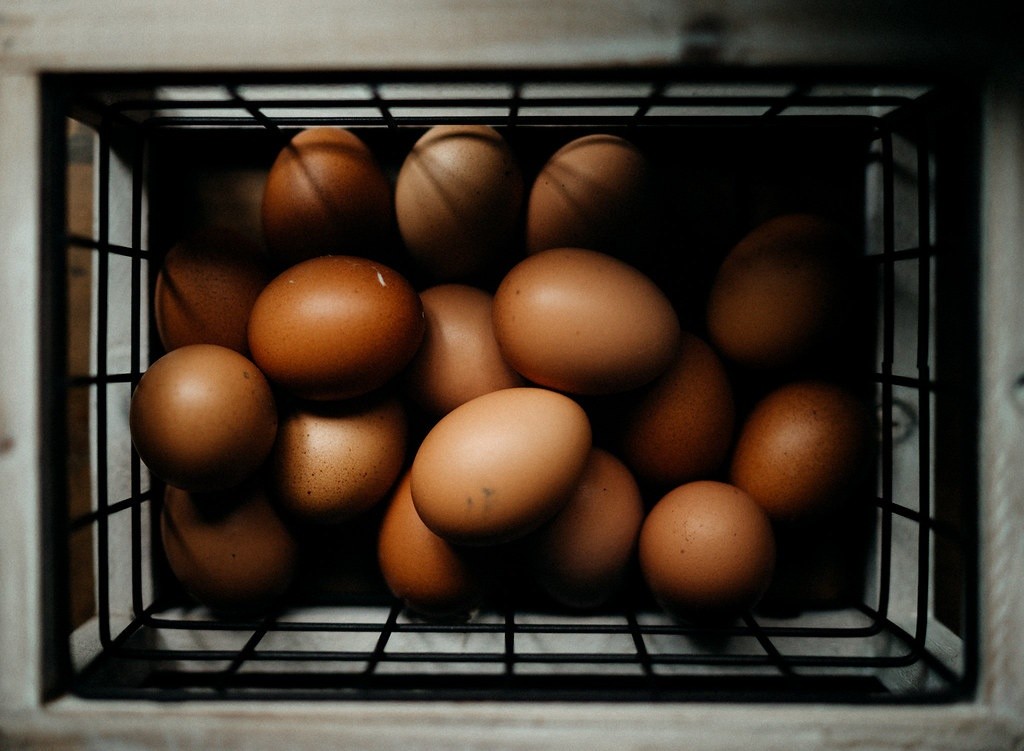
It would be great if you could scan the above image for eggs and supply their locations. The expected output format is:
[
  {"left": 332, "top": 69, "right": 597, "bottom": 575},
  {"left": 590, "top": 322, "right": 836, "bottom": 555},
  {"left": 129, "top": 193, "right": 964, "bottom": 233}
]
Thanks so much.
[{"left": 125, "top": 124, "right": 861, "bottom": 618}]
